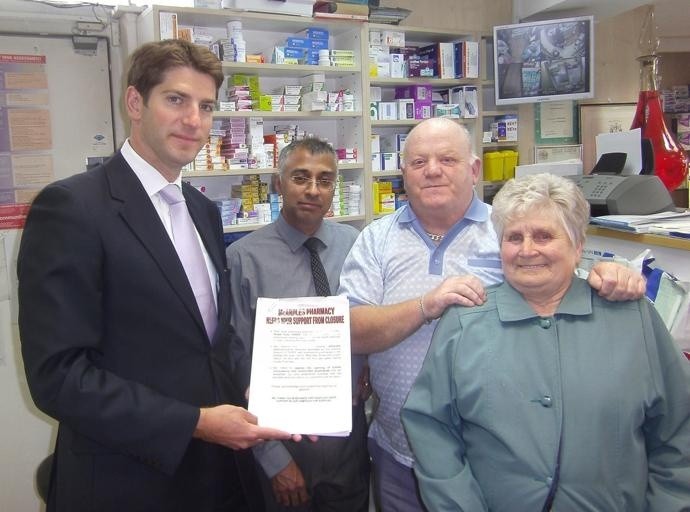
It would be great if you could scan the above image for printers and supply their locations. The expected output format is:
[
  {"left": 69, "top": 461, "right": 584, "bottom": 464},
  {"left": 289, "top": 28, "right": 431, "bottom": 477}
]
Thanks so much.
[{"left": 560, "top": 138, "right": 674, "bottom": 217}]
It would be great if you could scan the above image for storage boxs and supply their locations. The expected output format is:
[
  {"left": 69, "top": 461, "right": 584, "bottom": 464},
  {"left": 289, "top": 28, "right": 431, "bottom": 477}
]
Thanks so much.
[
  {"left": 483, "top": 148, "right": 504, "bottom": 181},
  {"left": 499, "top": 149, "right": 518, "bottom": 182}
]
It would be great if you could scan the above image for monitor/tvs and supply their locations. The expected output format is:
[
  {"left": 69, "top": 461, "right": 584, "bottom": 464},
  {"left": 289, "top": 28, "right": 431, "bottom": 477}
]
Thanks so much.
[{"left": 493, "top": 15, "right": 594, "bottom": 105}]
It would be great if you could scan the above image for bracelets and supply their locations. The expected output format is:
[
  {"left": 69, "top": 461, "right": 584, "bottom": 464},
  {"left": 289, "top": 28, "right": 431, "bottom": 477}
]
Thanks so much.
[{"left": 420, "top": 295, "right": 432, "bottom": 325}]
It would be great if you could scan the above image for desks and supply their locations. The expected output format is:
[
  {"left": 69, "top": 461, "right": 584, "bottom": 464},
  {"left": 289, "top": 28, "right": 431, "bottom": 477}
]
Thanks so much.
[{"left": 576, "top": 205, "right": 690, "bottom": 367}]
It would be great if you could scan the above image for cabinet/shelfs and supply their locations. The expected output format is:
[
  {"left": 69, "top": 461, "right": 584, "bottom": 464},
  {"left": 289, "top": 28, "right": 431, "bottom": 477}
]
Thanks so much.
[
  {"left": 364, "top": 22, "right": 518, "bottom": 207},
  {"left": 138, "top": 4, "right": 369, "bottom": 233}
]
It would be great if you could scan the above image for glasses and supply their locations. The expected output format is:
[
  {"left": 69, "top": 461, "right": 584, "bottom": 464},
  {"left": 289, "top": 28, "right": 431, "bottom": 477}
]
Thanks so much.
[{"left": 290, "top": 175, "right": 336, "bottom": 191}]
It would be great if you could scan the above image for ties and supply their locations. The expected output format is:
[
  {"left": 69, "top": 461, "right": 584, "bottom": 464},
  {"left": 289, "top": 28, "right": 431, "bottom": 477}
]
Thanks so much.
[
  {"left": 160, "top": 185, "right": 218, "bottom": 343},
  {"left": 304, "top": 240, "right": 331, "bottom": 296}
]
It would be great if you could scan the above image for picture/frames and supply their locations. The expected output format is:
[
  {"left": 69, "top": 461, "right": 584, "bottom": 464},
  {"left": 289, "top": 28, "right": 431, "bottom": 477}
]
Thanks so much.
[
  {"left": 577, "top": 102, "right": 642, "bottom": 177},
  {"left": 534, "top": 143, "right": 584, "bottom": 164}
]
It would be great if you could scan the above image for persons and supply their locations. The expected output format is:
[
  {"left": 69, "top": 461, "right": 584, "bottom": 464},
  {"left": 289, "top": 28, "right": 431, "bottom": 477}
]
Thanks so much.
[
  {"left": 226, "top": 138, "right": 373, "bottom": 512},
  {"left": 336, "top": 116, "right": 646, "bottom": 512},
  {"left": 400, "top": 173, "right": 690, "bottom": 512},
  {"left": 17, "top": 40, "right": 319, "bottom": 512}
]
